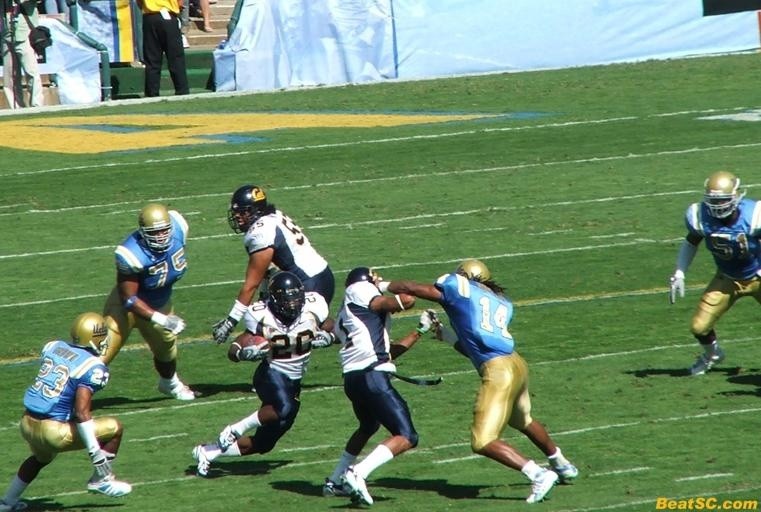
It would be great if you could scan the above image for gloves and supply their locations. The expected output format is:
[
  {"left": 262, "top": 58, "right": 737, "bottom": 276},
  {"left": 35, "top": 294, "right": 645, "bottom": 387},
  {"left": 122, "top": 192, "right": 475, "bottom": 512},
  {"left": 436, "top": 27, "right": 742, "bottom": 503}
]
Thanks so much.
[
  {"left": 430, "top": 318, "right": 451, "bottom": 342},
  {"left": 239, "top": 341, "right": 271, "bottom": 362},
  {"left": 162, "top": 315, "right": 186, "bottom": 335},
  {"left": 311, "top": 330, "right": 333, "bottom": 348},
  {"left": 212, "top": 315, "right": 239, "bottom": 346},
  {"left": 415, "top": 309, "right": 437, "bottom": 335},
  {"left": 670, "top": 274, "right": 685, "bottom": 304}
]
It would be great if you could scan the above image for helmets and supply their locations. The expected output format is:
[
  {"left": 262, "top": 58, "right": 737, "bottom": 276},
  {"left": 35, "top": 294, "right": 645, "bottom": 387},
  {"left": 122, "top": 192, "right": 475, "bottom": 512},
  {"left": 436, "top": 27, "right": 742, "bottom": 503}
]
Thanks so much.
[
  {"left": 703, "top": 171, "right": 747, "bottom": 219},
  {"left": 139, "top": 204, "right": 174, "bottom": 254},
  {"left": 71, "top": 312, "right": 112, "bottom": 356},
  {"left": 345, "top": 267, "right": 374, "bottom": 287},
  {"left": 228, "top": 186, "right": 267, "bottom": 234},
  {"left": 456, "top": 258, "right": 491, "bottom": 283},
  {"left": 268, "top": 272, "right": 305, "bottom": 320}
]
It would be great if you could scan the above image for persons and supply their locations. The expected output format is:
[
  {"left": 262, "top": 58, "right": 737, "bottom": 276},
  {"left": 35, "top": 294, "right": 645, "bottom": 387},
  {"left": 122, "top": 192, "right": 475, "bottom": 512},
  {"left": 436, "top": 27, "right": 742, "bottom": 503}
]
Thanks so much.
[
  {"left": 0, "top": 311, "right": 132, "bottom": 511},
  {"left": 322, "top": 266, "right": 438, "bottom": 505},
  {"left": 210, "top": 185, "right": 335, "bottom": 394},
  {"left": 136, "top": 0, "right": 189, "bottom": 99},
  {"left": 191, "top": 270, "right": 340, "bottom": 476},
  {"left": 98, "top": 203, "right": 195, "bottom": 403},
  {"left": 38, "top": 0, "right": 67, "bottom": 14},
  {"left": 176, "top": 0, "right": 214, "bottom": 50},
  {"left": 669, "top": 168, "right": 761, "bottom": 376},
  {"left": 374, "top": 259, "right": 579, "bottom": 504}
]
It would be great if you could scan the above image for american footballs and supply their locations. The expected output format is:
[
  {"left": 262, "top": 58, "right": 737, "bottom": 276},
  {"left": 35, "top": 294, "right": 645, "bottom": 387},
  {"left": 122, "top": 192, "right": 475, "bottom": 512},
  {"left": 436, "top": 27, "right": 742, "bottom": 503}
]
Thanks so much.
[{"left": 242, "top": 336, "right": 269, "bottom": 358}]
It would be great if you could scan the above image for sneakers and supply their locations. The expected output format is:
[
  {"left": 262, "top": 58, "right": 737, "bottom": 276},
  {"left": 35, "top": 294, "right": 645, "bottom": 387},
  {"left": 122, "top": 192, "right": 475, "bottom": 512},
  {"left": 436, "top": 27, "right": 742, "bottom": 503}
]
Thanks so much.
[
  {"left": 0, "top": 378, "right": 580, "bottom": 511},
  {"left": 691, "top": 347, "right": 726, "bottom": 376}
]
